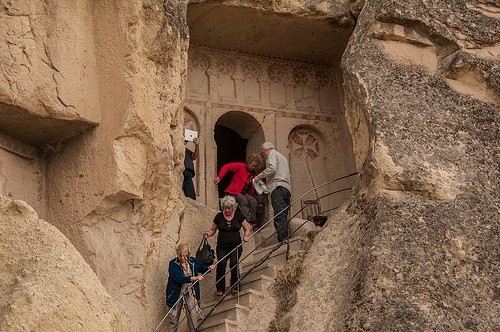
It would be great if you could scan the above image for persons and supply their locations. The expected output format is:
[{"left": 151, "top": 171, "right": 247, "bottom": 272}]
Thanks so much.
[
  {"left": 203, "top": 195, "right": 252, "bottom": 297},
  {"left": 213, "top": 152, "right": 265, "bottom": 234},
  {"left": 181, "top": 137, "right": 200, "bottom": 200},
  {"left": 255, "top": 142, "right": 293, "bottom": 244},
  {"left": 165, "top": 241, "right": 216, "bottom": 332}
]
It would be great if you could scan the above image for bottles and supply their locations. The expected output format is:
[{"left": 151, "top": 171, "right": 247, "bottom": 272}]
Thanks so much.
[{"left": 257, "top": 179, "right": 270, "bottom": 194}]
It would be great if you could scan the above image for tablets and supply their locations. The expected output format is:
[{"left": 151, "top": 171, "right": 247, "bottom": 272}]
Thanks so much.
[{"left": 185, "top": 129, "right": 198, "bottom": 142}]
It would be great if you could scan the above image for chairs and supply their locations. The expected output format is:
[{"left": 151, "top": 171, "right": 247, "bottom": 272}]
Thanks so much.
[{"left": 301, "top": 198, "right": 328, "bottom": 227}]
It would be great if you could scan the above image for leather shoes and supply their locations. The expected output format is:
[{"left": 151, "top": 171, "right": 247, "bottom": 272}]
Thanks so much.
[
  {"left": 231, "top": 289, "right": 237, "bottom": 293},
  {"left": 214, "top": 291, "right": 225, "bottom": 296}
]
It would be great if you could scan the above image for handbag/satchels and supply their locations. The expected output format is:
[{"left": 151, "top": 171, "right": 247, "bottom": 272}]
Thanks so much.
[{"left": 196, "top": 237, "right": 214, "bottom": 266}]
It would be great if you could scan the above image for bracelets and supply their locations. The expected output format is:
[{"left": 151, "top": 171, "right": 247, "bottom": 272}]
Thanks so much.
[{"left": 244, "top": 234, "right": 249, "bottom": 237}]
